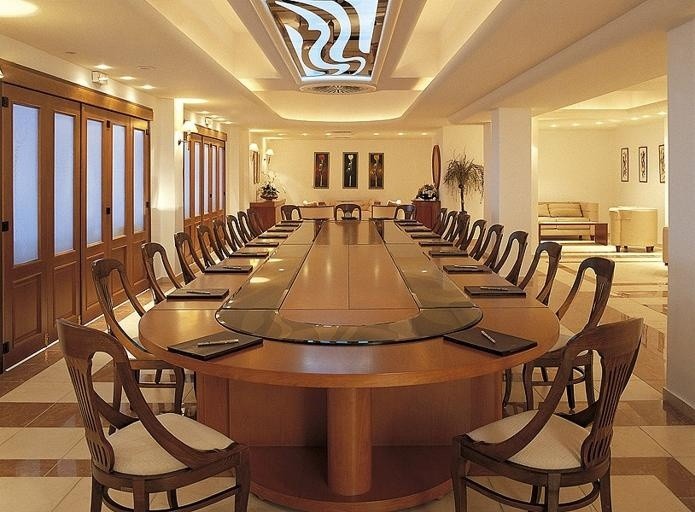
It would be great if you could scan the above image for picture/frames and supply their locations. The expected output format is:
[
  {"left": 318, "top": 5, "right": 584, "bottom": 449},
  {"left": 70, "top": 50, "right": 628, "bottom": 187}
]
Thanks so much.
[
  {"left": 431, "top": 144, "right": 441, "bottom": 189},
  {"left": 312, "top": 150, "right": 330, "bottom": 190},
  {"left": 620, "top": 148, "right": 629, "bottom": 182},
  {"left": 659, "top": 145, "right": 666, "bottom": 183},
  {"left": 638, "top": 146, "right": 648, "bottom": 183},
  {"left": 368, "top": 152, "right": 386, "bottom": 191},
  {"left": 341, "top": 152, "right": 359, "bottom": 189}
]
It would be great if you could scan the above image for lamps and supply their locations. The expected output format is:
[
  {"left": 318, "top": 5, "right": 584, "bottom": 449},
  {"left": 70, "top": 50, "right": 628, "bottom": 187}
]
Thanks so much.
[
  {"left": 249, "top": 143, "right": 258, "bottom": 152},
  {"left": 181, "top": 120, "right": 198, "bottom": 143},
  {"left": 265, "top": 149, "right": 273, "bottom": 163}
]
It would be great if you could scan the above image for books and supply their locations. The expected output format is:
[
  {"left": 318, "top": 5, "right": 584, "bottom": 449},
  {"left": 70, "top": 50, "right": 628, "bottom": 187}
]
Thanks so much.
[
  {"left": 464, "top": 285, "right": 527, "bottom": 298},
  {"left": 394, "top": 219, "right": 454, "bottom": 247},
  {"left": 229, "top": 219, "right": 304, "bottom": 257},
  {"left": 429, "top": 249, "right": 468, "bottom": 258},
  {"left": 166, "top": 330, "right": 263, "bottom": 360},
  {"left": 204, "top": 264, "right": 253, "bottom": 273},
  {"left": 442, "top": 264, "right": 492, "bottom": 274},
  {"left": 166, "top": 287, "right": 229, "bottom": 299},
  {"left": 443, "top": 326, "right": 537, "bottom": 357}
]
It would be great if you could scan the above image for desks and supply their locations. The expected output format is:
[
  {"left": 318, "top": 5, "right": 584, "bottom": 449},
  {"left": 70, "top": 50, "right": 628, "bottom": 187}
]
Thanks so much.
[{"left": 608, "top": 205, "right": 658, "bottom": 252}]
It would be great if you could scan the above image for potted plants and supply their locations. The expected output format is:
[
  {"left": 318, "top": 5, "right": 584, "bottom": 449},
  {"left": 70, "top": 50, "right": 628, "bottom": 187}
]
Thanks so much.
[
  {"left": 256, "top": 174, "right": 286, "bottom": 202},
  {"left": 444, "top": 155, "right": 486, "bottom": 239},
  {"left": 417, "top": 185, "right": 438, "bottom": 200}
]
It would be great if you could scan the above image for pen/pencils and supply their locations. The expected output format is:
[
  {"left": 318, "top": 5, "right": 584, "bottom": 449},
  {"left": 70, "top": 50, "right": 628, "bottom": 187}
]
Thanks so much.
[
  {"left": 454, "top": 265, "right": 477, "bottom": 268},
  {"left": 480, "top": 331, "right": 496, "bottom": 344},
  {"left": 256, "top": 243, "right": 270, "bottom": 244},
  {"left": 241, "top": 253, "right": 258, "bottom": 254},
  {"left": 222, "top": 266, "right": 242, "bottom": 269},
  {"left": 198, "top": 339, "right": 239, "bottom": 346},
  {"left": 440, "top": 251, "right": 450, "bottom": 253},
  {"left": 187, "top": 291, "right": 210, "bottom": 294},
  {"left": 480, "top": 287, "right": 509, "bottom": 291}
]
